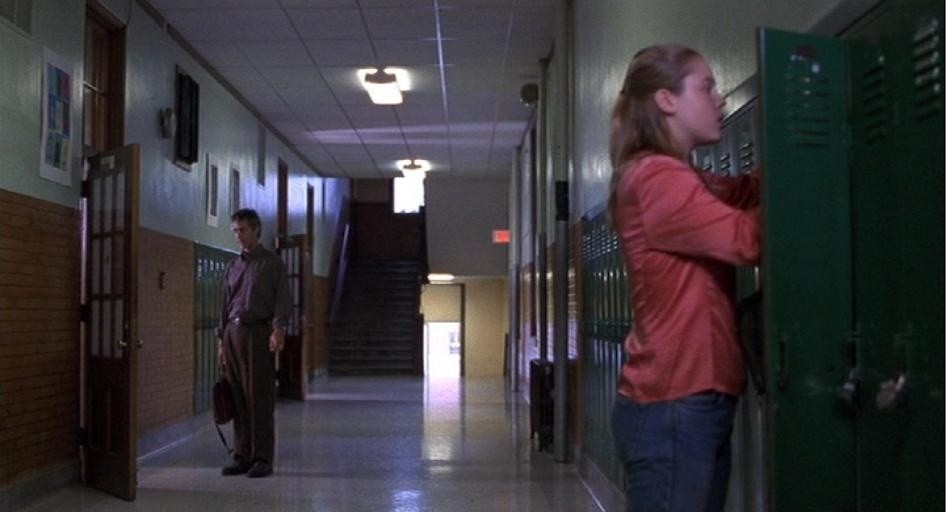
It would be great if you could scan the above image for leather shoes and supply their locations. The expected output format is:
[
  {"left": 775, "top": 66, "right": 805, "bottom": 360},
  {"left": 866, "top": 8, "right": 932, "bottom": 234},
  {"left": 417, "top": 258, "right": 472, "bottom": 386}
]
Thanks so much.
[{"left": 222, "top": 461, "right": 273, "bottom": 477}]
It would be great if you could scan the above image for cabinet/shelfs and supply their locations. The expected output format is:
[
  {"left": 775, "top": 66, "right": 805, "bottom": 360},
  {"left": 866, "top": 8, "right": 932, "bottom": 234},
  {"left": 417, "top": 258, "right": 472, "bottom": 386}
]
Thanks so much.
[{"left": 571, "top": 1, "right": 946, "bottom": 512}]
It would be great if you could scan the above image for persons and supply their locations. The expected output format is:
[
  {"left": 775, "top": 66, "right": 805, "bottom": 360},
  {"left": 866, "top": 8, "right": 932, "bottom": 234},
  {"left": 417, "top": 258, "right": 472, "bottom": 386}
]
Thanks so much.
[
  {"left": 606, "top": 43, "right": 764, "bottom": 512},
  {"left": 214, "top": 208, "right": 295, "bottom": 477}
]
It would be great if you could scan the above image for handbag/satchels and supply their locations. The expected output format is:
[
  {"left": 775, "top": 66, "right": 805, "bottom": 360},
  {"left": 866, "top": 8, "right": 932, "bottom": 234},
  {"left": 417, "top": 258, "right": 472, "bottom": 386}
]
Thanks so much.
[{"left": 212, "top": 375, "right": 234, "bottom": 424}]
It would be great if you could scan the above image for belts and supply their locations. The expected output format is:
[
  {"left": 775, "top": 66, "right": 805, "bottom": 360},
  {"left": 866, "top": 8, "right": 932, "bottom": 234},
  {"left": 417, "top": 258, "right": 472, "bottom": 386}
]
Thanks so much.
[{"left": 233, "top": 318, "right": 265, "bottom": 324}]
